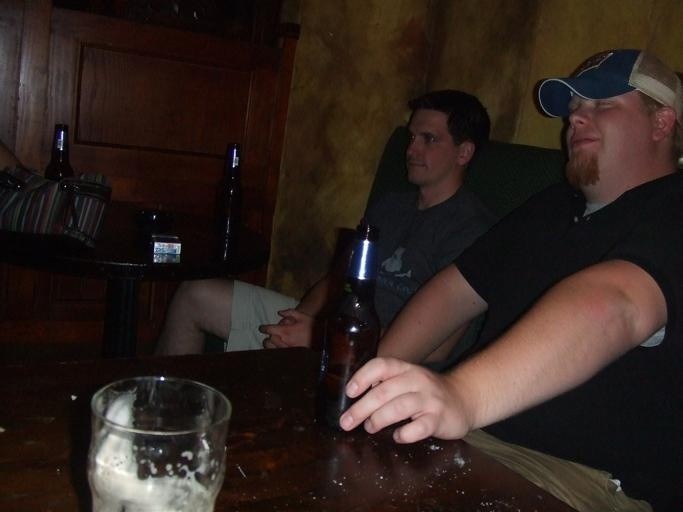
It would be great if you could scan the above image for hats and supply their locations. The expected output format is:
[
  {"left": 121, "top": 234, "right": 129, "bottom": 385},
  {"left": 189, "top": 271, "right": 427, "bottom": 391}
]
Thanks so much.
[{"left": 536, "top": 48, "right": 681, "bottom": 129}]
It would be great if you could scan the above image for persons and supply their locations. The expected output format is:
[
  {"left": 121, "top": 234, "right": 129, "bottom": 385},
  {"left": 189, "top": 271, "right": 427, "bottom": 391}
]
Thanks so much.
[
  {"left": 337, "top": 46, "right": 682, "bottom": 512},
  {"left": 147, "top": 84, "right": 504, "bottom": 368}
]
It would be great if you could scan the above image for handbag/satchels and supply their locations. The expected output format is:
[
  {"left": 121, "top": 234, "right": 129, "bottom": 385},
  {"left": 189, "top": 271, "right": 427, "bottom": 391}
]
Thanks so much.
[{"left": 0, "top": 163, "right": 111, "bottom": 272}]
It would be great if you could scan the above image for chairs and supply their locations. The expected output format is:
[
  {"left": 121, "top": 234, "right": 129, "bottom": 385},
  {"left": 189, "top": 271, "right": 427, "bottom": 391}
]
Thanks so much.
[{"left": 362, "top": 127, "right": 566, "bottom": 369}]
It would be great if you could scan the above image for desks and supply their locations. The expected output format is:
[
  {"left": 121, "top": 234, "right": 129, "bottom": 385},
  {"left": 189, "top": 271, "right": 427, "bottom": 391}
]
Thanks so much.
[
  {"left": 0, "top": 346, "right": 576, "bottom": 512},
  {"left": 60, "top": 237, "right": 212, "bottom": 358}
]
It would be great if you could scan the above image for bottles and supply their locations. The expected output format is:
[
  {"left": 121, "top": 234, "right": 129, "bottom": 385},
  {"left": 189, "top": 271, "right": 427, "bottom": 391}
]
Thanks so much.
[
  {"left": 311, "top": 221, "right": 382, "bottom": 432},
  {"left": 212, "top": 142, "right": 244, "bottom": 265},
  {"left": 43, "top": 123, "right": 73, "bottom": 181}
]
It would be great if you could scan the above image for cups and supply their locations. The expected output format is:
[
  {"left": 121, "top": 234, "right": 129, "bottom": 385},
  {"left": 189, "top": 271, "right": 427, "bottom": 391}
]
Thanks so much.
[{"left": 86, "top": 375, "right": 233, "bottom": 512}]
